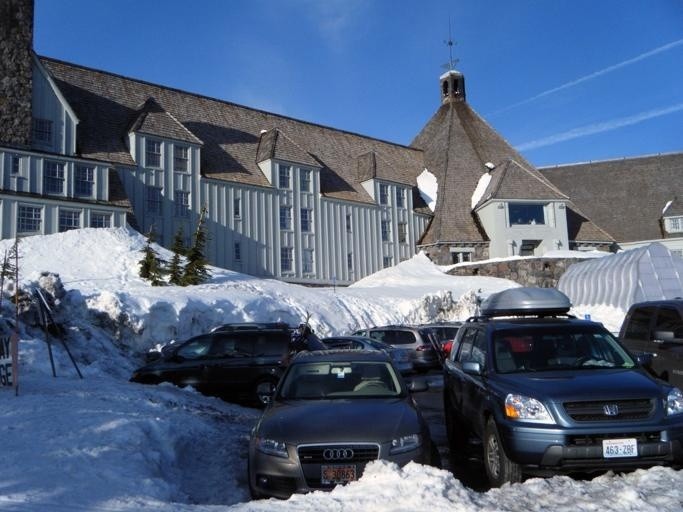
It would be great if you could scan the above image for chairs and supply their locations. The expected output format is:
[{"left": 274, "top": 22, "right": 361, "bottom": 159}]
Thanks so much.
[
  {"left": 355, "top": 366, "right": 386, "bottom": 392},
  {"left": 490, "top": 341, "right": 517, "bottom": 370},
  {"left": 295, "top": 378, "right": 325, "bottom": 401}
]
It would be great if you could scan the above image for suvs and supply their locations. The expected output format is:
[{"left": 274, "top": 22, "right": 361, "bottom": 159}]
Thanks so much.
[
  {"left": 443, "top": 287, "right": 683, "bottom": 488},
  {"left": 210, "top": 322, "right": 290, "bottom": 334},
  {"left": 128, "top": 328, "right": 299, "bottom": 407},
  {"left": 352, "top": 322, "right": 468, "bottom": 374},
  {"left": 617, "top": 298, "right": 683, "bottom": 392}
]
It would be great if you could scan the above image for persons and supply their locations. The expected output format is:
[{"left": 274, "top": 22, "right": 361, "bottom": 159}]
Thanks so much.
[{"left": 574, "top": 336, "right": 602, "bottom": 364}]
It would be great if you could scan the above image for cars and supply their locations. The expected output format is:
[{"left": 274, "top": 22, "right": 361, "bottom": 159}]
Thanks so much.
[
  {"left": 247, "top": 347, "right": 441, "bottom": 502},
  {"left": 320, "top": 337, "right": 418, "bottom": 382}
]
